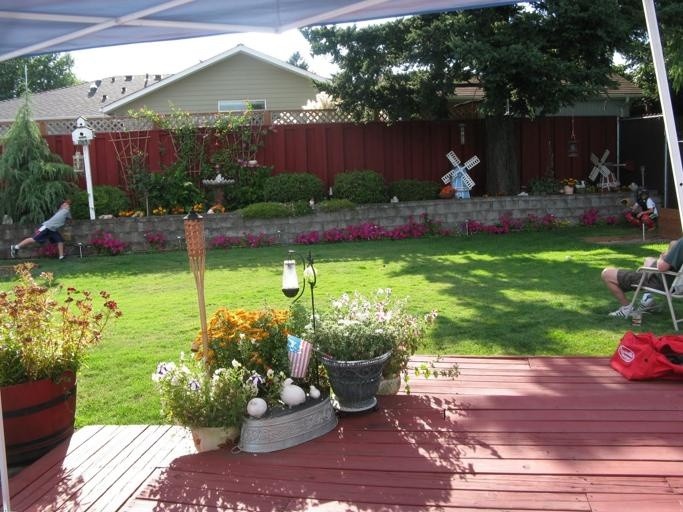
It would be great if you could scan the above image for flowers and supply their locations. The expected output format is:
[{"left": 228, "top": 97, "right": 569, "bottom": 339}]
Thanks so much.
[{"left": 1, "top": 259, "right": 123, "bottom": 382}]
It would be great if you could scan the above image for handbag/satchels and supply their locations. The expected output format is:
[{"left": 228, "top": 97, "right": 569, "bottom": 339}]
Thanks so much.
[{"left": 610, "top": 332, "right": 682, "bottom": 381}]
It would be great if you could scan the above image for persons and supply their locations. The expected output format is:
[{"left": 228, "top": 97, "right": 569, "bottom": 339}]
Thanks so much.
[
  {"left": 600, "top": 237, "right": 683, "bottom": 319},
  {"left": 625, "top": 188, "right": 659, "bottom": 233},
  {"left": 9, "top": 199, "right": 74, "bottom": 261}
]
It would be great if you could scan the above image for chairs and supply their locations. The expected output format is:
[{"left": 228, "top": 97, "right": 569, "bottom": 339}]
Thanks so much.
[{"left": 624, "top": 265, "right": 683, "bottom": 331}]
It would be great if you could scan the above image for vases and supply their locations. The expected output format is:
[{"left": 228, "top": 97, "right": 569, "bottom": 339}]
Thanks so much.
[
  {"left": 0, "top": 359, "right": 76, "bottom": 472},
  {"left": 190, "top": 421, "right": 241, "bottom": 455},
  {"left": 239, "top": 398, "right": 338, "bottom": 452}
]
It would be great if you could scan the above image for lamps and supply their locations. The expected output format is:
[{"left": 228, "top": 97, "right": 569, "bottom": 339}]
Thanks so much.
[{"left": 283, "top": 249, "right": 319, "bottom": 387}]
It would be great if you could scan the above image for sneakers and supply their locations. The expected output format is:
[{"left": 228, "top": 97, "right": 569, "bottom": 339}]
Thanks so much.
[
  {"left": 59, "top": 256, "right": 65, "bottom": 260},
  {"left": 11, "top": 245, "right": 20, "bottom": 258},
  {"left": 609, "top": 305, "right": 635, "bottom": 318},
  {"left": 637, "top": 293, "right": 657, "bottom": 312}
]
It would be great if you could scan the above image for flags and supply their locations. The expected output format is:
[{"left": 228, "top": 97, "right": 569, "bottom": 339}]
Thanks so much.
[{"left": 283, "top": 333, "right": 314, "bottom": 380}]
